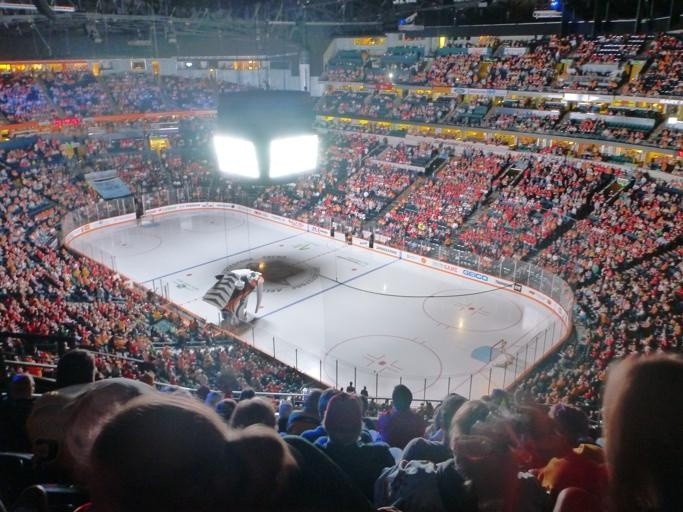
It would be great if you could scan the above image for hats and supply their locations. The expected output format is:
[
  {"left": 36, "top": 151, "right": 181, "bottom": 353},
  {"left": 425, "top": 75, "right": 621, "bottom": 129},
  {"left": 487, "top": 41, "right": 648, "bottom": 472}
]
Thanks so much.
[
  {"left": 321, "top": 392, "right": 363, "bottom": 434},
  {"left": 25, "top": 378, "right": 297, "bottom": 510}
]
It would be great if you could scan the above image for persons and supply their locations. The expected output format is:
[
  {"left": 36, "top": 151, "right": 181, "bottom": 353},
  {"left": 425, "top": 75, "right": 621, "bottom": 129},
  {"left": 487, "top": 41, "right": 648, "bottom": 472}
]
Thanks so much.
[{"left": 408, "top": 250, "right": 683, "bottom": 333}]
